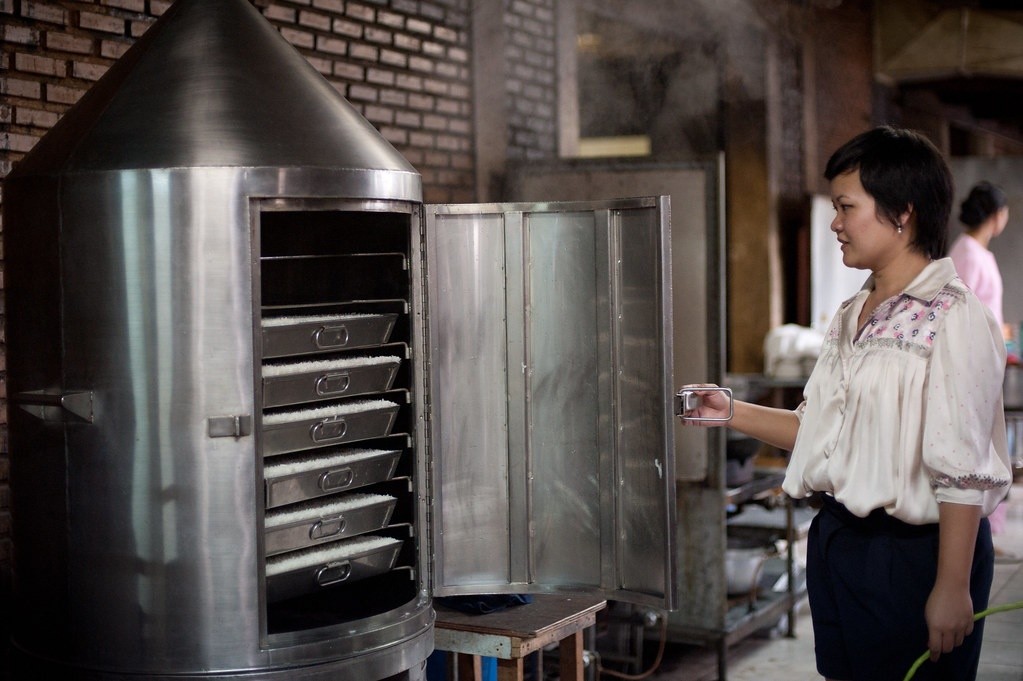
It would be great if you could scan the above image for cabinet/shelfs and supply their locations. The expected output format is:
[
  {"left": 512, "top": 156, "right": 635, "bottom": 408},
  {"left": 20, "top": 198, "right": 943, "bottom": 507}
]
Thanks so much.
[{"left": 599, "top": 461, "right": 821, "bottom": 681}]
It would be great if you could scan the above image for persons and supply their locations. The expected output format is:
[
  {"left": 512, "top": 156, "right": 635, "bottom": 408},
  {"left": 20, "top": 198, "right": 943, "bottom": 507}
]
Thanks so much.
[
  {"left": 945, "top": 178, "right": 1009, "bottom": 332},
  {"left": 680, "top": 126, "right": 1017, "bottom": 681}
]
